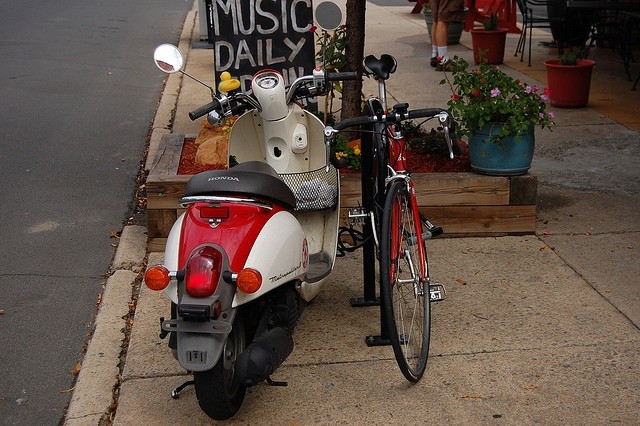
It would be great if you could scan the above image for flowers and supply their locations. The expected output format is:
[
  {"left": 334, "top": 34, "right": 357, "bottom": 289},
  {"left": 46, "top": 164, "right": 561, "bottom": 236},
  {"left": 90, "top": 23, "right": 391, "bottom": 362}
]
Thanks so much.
[{"left": 436, "top": 54, "right": 555, "bottom": 151}]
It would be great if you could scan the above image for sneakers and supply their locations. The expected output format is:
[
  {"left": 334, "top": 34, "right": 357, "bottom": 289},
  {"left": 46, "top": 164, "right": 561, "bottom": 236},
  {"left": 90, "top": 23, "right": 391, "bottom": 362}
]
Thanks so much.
[
  {"left": 437, "top": 59, "right": 465, "bottom": 71},
  {"left": 431, "top": 55, "right": 438, "bottom": 66}
]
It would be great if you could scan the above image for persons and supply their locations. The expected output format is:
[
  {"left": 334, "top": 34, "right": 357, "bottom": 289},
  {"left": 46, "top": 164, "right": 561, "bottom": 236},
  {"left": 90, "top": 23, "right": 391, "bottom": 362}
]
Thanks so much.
[{"left": 428, "top": 0, "right": 466, "bottom": 71}]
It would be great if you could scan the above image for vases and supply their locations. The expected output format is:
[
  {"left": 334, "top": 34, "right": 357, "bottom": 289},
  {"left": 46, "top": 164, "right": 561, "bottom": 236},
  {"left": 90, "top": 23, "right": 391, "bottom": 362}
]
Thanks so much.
[
  {"left": 424, "top": 7, "right": 469, "bottom": 45},
  {"left": 467, "top": 116, "right": 535, "bottom": 176}
]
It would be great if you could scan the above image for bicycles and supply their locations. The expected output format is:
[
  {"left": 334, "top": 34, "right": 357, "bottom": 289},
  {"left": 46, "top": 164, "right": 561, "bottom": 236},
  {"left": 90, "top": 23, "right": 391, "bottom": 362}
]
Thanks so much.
[{"left": 330, "top": 53, "right": 455, "bottom": 383}]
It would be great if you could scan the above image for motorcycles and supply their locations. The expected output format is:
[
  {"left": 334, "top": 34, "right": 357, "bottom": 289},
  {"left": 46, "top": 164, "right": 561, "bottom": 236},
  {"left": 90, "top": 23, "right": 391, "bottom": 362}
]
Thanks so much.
[{"left": 145, "top": 44, "right": 358, "bottom": 420}]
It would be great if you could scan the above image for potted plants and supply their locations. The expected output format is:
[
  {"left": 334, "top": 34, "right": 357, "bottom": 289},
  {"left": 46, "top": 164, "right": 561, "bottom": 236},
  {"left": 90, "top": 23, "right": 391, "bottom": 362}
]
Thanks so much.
[
  {"left": 469, "top": 15, "right": 508, "bottom": 64},
  {"left": 544, "top": 54, "right": 595, "bottom": 109}
]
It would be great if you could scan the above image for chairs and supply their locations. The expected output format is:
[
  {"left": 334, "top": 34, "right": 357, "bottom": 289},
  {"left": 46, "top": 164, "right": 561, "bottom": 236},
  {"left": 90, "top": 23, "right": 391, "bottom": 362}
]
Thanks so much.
[{"left": 514, "top": 0, "right": 567, "bottom": 67}]
